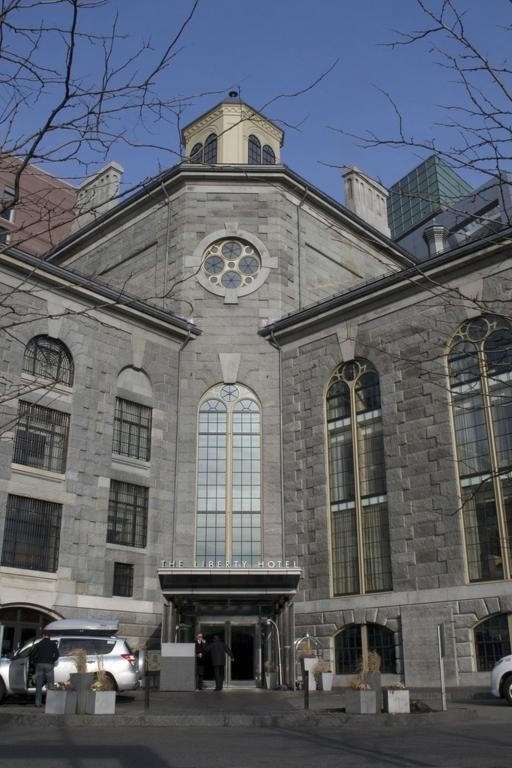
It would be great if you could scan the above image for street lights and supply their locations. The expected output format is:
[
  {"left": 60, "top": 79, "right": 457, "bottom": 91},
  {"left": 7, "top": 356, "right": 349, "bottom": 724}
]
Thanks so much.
[{"left": 263, "top": 618, "right": 283, "bottom": 691}]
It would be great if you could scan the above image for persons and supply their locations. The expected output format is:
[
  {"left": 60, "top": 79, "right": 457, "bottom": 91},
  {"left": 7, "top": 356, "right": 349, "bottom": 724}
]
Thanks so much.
[
  {"left": 197, "top": 634, "right": 235, "bottom": 692},
  {"left": 28, "top": 629, "right": 60, "bottom": 707},
  {"left": 194, "top": 632, "right": 208, "bottom": 691}
]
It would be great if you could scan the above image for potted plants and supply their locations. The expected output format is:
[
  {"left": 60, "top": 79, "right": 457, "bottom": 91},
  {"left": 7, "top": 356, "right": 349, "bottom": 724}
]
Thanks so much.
[
  {"left": 344, "top": 649, "right": 411, "bottom": 714},
  {"left": 44, "top": 648, "right": 117, "bottom": 715},
  {"left": 299, "top": 643, "right": 333, "bottom": 691},
  {"left": 264, "top": 661, "right": 277, "bottom": 689}
]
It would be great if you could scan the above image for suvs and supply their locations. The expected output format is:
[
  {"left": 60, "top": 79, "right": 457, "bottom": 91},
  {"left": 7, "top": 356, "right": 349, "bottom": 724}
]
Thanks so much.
[
  {"left": 0, "top": 617, "right": 148, "bottom": 703},
  {"left": 490, "top": 654, "right": 511, "bottom": 707}
]
